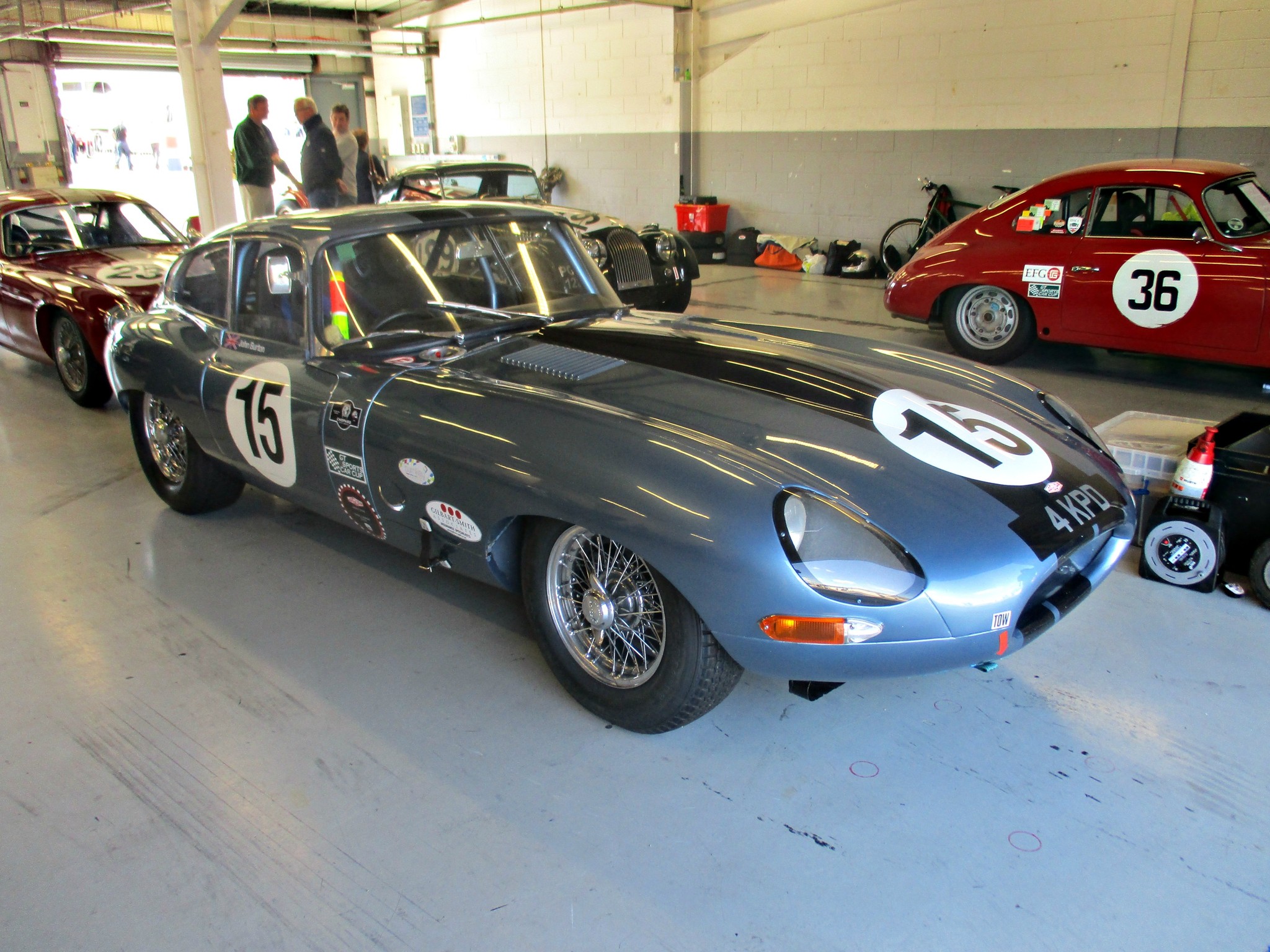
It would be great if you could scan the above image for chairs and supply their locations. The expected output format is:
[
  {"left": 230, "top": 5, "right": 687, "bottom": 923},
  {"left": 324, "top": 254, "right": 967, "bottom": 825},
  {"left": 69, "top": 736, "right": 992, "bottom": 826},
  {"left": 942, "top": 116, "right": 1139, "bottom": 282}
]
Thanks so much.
[
  {"left": 1116, "top": 192, "right": 1154, "bottom": 237},
  {"left": 352, "top": 235, "right": 468, "bottom": 329},
  {"left": 252, "top": 240, "right": 379, "bottom": 346},
  {"left": 10, "top": 224, "right": 54, "bottom": 256}
]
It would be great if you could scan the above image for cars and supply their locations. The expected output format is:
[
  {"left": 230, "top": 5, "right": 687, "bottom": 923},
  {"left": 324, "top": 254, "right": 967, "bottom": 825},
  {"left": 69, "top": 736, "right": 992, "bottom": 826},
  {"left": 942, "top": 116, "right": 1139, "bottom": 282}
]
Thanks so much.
[
  {"left": 883, "top": 159, "right": 1270, "bottom": 380},
  {"left": 101, "top": 198, "right": 1141, "bottom": 734},
  {"left": 368, "top": 160, "right": 701, "bottom": 312},
  {"left": 0, "top": 187, "right": 222, "bottom": 409}
]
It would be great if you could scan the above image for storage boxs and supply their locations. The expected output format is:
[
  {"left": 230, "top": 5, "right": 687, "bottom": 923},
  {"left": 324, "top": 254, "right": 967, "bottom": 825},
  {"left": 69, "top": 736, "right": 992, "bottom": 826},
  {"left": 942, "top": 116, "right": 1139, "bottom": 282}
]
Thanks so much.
[
  {"left": 1093, "top": 409, "right": 1219, "bottom": 548},
  {"left": 673, "top": 203, "right": 730, "bottom": 233}
]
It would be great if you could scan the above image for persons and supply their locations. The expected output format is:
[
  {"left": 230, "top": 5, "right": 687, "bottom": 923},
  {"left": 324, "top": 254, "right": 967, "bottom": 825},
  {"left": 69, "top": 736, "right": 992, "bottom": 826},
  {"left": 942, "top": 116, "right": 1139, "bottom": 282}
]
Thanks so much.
[
  {"left": 113, "top": 123, "right": 133, "bottom": 171},
  {"left": 351, "top": 128, "right": 388, "bottom": 204},
  {"left": 233, "top": 94, "right": 306, "bottom": 222},
  {"left": 328, "top": 105, "right": 358, "bottom": 208},
  {"left": 67, "top": 126, "right": 78, "bottom": 165},
  {"left": 293, "top": 102, "right": 348, "bottom": 209}
]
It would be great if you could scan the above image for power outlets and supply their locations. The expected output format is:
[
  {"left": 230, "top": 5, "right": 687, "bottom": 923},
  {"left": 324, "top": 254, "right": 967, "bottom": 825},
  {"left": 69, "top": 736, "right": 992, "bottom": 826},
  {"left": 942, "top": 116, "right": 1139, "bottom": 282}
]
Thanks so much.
[{"left": 46, "top": 154, "right": 57, "bottom": 163}]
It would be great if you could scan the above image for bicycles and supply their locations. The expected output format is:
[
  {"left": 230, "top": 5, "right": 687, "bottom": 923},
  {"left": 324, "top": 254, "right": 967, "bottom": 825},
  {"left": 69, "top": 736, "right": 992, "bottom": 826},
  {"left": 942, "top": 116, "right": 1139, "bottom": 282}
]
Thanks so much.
[{"left": 878, "top": 174, "right": 1022, "bottom": 277}]
